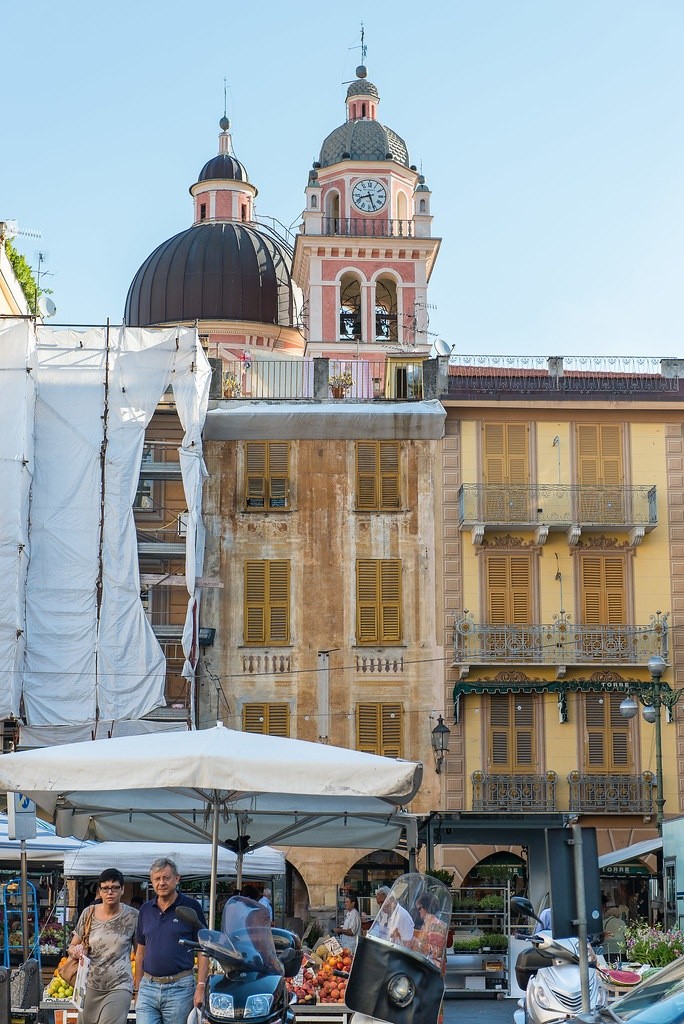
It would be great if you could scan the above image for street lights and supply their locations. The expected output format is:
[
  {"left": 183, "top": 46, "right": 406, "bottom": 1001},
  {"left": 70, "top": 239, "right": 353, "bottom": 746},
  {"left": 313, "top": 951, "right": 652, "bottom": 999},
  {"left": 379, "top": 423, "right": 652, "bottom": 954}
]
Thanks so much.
[{"left": 618, "top": 656, "right": 669, "bottom": 911}]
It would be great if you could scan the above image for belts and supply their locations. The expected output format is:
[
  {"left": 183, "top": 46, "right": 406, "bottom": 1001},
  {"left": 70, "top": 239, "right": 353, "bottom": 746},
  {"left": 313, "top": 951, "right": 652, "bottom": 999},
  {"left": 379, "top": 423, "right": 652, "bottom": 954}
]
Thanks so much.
[{"left": 143, "top": 969, "right": 193, "bottom": 983}]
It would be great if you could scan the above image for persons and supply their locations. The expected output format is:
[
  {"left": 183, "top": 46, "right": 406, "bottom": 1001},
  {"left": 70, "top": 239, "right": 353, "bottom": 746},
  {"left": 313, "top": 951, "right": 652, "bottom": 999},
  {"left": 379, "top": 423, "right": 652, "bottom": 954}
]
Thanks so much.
[
  {"left": 415, "top": 893, "right": 452, "bottom": 1024},
  {"left": 133, "top": 857, "right": 209, "bottom": 1024},
  {"left": 68, "top": 869, "right": 139, "bottom": 1024},
  {"left": 602, "top": 907, "right": 627, "bottom": 964},
  {"left": 536, "top": 908, "right": 552, "bottom": 933},
  {"left": 333, "top": 896, "right": 361, "bottom": 955},
  {"left": 258, "top": 888, "right": 274, "bottom": 928},
  {"left": 375, "top": 886, "right": 414, "bottom": 946}
]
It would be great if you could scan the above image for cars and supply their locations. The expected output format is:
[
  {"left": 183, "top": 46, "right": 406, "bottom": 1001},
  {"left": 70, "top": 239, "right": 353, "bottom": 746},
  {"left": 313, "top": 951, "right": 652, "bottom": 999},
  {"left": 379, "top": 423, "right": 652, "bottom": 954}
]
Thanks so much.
[{"left": 558, "top": 953, "right": 684, "bottom": 1024}]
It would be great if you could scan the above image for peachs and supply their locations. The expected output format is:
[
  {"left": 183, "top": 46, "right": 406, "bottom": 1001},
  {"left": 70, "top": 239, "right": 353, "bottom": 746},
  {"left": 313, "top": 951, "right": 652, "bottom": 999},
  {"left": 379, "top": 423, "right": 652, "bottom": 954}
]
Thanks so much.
[{"left": 285, "top": 945, "right": 353, "bottom": 1004}]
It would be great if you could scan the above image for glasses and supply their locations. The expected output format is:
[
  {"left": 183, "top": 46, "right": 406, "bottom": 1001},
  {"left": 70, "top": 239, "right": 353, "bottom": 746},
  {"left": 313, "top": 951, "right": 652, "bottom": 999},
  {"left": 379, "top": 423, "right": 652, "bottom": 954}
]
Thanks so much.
[
  {"left": 419, "top": 907, "right": 423, "bottom": 910},
  {"left": 100, "top": 886, "right": 121, "bottom": 893}
]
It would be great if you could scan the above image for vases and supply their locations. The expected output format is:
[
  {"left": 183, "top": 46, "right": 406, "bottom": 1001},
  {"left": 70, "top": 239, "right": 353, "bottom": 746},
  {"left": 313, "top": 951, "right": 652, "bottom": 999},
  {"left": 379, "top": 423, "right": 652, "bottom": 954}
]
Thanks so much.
[
  {"left": 331, "top": 385, "right": 344, "bottom": 398},
  {"left": 224, "top": 389, "right": 239, "bottom": 398},
  {"left": 641, "top": 967, "right": 663, "bottom": 980}
]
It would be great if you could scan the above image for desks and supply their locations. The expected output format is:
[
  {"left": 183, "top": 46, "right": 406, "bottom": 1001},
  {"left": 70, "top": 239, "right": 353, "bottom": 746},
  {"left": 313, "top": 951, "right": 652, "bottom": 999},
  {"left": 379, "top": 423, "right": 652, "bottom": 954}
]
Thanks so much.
[{"left": 36, "top": 1002, "right": 356, "bottom": 1024}]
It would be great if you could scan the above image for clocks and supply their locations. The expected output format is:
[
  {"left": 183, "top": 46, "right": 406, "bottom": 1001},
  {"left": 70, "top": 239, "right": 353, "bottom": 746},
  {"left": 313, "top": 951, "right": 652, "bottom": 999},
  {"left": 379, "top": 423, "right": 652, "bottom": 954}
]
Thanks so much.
[{"left": 353, "top": 180, "right": 386, "bottom": 212}]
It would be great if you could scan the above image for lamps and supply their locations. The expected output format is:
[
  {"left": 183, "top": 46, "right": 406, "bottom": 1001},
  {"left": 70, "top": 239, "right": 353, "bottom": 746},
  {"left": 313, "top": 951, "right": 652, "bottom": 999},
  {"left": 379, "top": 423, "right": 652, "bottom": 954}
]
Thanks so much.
[{"left": 431, "top": 714, "right": 451, "bottom": 776}]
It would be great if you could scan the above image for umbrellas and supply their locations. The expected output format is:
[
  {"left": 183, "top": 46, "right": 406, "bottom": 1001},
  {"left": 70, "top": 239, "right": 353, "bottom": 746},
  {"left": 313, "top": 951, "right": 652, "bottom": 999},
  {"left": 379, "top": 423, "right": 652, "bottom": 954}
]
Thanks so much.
[{"left": 0, "top": 721, "right": 423, "bottom": 930}]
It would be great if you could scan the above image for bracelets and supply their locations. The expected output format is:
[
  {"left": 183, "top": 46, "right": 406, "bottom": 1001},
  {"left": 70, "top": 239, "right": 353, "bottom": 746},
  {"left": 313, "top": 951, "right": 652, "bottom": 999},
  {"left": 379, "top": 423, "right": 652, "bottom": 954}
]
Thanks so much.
[{"left": 133, "top": 989, "right": 138, "bottom": 993}]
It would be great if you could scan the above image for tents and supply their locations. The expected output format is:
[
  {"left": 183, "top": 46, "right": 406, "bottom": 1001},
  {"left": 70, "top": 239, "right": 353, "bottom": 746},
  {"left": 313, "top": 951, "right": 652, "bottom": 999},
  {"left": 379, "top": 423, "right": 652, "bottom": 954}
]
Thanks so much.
[{"left": 63, "top": 841, "right": 286, "bottom": 923}]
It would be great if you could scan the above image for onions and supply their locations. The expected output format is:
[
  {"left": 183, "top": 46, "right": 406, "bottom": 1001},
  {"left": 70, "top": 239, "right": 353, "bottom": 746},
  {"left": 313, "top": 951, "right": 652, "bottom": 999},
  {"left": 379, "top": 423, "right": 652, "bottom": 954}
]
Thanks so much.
[{"left": 39, "top": 944, "right": 60, "bottom": 955}]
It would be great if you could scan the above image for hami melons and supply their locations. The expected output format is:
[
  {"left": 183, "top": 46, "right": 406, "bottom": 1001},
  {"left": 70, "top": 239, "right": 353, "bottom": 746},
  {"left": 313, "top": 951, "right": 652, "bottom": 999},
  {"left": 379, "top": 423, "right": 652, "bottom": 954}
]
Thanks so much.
[{"left": 316, "top": 945, "right": 329, "bottom": 957}]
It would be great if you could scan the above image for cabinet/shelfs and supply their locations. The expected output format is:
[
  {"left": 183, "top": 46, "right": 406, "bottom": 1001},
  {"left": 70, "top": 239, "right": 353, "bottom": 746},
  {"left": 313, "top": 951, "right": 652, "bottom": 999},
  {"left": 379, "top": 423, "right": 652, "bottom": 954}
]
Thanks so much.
[{"left": 427, "top": 880, "right": 512, "bottom": 995}]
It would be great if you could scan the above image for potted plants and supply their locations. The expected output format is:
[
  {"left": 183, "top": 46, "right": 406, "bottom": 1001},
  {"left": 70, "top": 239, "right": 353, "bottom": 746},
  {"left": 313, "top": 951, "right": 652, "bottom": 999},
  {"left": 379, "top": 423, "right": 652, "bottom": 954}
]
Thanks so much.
[
  {"left": 425, "top": 865, "right": 511, "bottom": 887},
  {"left": 454, "top": 936, "right": 509, "bottom": 953},
  {"left": 435, "top": 891, "right": 505, "bottom": 912}
]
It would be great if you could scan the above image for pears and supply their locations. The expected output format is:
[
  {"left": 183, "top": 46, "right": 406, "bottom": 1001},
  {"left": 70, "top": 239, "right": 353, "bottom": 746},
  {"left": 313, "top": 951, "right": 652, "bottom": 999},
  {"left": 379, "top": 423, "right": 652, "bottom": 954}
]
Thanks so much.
[{"left": 54, "top": 956, "right": 70, "bottom": 977}]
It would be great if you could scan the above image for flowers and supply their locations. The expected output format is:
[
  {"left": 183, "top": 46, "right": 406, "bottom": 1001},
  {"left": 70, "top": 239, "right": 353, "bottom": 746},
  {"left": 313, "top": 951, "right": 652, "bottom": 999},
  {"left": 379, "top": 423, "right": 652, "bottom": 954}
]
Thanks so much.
[
  {"left": 225, "top": 373, "right": 243, "bottom": 390},
  {"left": 328, "top": 372, "right": 353, "bottom": 387},
  {"left": 616, "top": 913, "right": 684, "bottom": 964}
]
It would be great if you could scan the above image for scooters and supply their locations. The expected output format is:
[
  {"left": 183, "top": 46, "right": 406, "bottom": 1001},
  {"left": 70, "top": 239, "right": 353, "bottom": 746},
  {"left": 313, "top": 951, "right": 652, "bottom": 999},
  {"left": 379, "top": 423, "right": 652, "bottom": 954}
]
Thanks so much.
[{"left": 509, "top": 896, "right": 613, "bottom": 1024}]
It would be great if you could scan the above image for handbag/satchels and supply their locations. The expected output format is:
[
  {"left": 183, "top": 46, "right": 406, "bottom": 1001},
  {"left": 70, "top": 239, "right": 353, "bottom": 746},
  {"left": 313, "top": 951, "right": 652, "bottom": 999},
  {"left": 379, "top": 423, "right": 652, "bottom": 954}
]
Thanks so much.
[{"left": 58, "top": 935, "right": 89, "bottom": 988}]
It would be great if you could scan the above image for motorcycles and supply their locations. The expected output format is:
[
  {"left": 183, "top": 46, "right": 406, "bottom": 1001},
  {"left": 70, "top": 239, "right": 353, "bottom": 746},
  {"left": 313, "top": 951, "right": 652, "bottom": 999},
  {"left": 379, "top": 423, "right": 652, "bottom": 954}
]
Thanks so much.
[
  {"left": 172, "top": 894, "right": 305, "bottom": 1024},
  {"left": 331, "top": 872, "right": 454, "bottom": 1024}
]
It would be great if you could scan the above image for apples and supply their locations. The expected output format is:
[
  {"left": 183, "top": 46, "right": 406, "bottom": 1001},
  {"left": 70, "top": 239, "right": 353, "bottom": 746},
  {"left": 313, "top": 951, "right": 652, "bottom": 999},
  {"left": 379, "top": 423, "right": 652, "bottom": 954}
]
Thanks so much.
[{"left": 47, "top": 976, "right": 73, "bottom": 998}]
